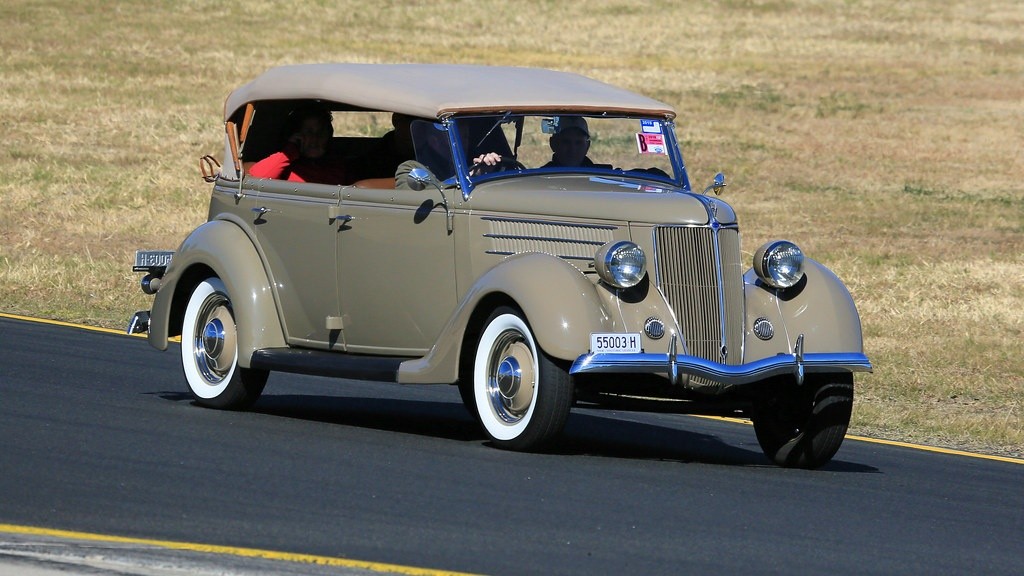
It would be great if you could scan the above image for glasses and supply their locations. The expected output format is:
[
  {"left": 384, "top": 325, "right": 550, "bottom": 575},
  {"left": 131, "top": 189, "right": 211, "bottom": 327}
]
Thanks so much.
[
  {"left": 301, "top": 128, "right": 328, "bottom": 137},
  {"left": 561, "top": 139, "right": 588, "bottom": 147}
]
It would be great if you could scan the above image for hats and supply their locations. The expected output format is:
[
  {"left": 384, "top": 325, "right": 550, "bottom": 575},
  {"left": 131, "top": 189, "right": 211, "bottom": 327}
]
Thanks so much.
[{"left": 556, "top": 116, "right": 590, "bottom": 136}]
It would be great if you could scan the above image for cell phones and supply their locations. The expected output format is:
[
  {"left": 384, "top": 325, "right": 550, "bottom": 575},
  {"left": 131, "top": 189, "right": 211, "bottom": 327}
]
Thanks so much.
[{"left": 296, "top": 139, "right": 303, "bottom": 153}]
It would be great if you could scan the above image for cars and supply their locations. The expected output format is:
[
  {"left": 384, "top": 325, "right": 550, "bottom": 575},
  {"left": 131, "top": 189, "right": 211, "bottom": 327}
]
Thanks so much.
[{"left": 127, "top": 60, "right": 876, "bottom": 469}]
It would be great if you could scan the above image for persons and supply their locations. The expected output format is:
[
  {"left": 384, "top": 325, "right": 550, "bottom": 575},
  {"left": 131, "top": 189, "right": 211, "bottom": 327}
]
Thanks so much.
[
  {"left": 541, "top": 116, "right": 595, "bottom": 168},
  {"left": 247, "top": 102, "right": 505, "bottom": 190}
]
